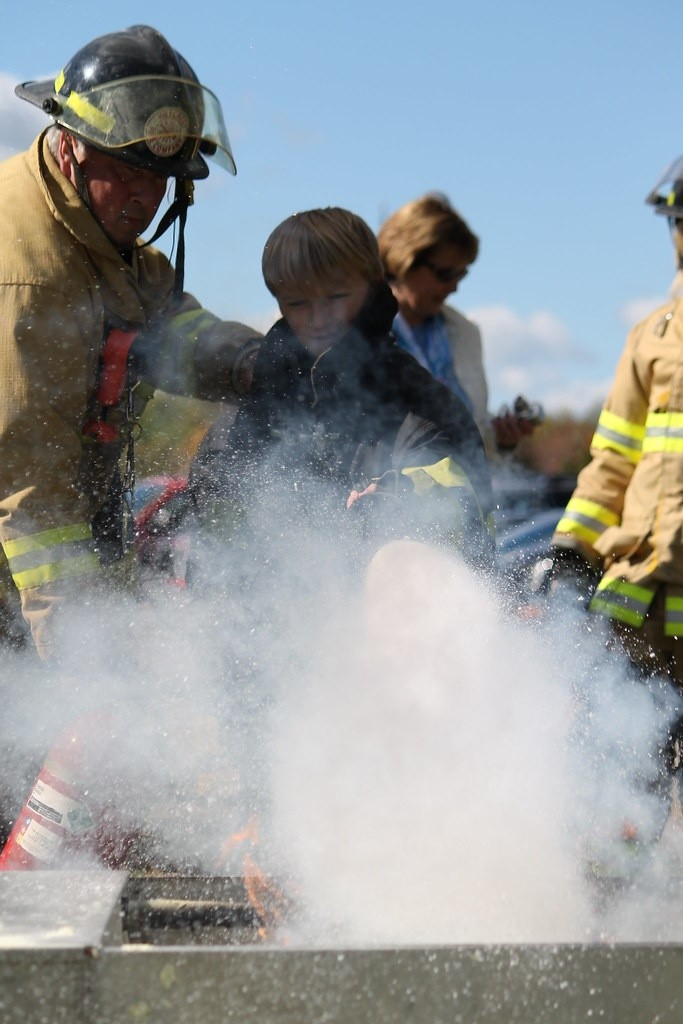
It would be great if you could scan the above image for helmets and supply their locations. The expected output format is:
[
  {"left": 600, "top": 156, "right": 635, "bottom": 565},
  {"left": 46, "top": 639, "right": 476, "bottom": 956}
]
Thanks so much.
[
  {"left": 644, "top": 153, "right": 683, "bottom": 222},
  {"left": 13, "top": 24, "right": 237, "bottom": 180}
]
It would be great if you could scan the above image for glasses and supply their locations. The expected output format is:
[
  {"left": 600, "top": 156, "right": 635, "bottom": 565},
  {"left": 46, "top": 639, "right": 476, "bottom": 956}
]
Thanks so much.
[{"left": 417, "top": 254, "right": 471, "bottom": 284}]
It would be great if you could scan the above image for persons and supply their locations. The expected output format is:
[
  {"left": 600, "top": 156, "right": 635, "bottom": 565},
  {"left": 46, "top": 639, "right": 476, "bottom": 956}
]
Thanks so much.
[
  {"left": 191, "top": 190, "right": 495, "bottom": 663},
  {"left": 530, "top": 156, "right": 683, "bottom": 942},
  {"left": 0, "top": 26, "right": 262, "bottom": 831}
]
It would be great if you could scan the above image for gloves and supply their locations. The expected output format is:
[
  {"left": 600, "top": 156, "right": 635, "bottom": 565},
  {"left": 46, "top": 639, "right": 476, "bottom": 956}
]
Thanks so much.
[{"left": 530, "top": 537, "right": 601, "bottom": 615}]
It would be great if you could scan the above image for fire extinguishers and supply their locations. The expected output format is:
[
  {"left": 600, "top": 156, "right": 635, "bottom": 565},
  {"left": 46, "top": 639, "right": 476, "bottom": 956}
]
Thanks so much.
[{"left": 0, "top": 685, "right": 136, "bottom": 871}]
